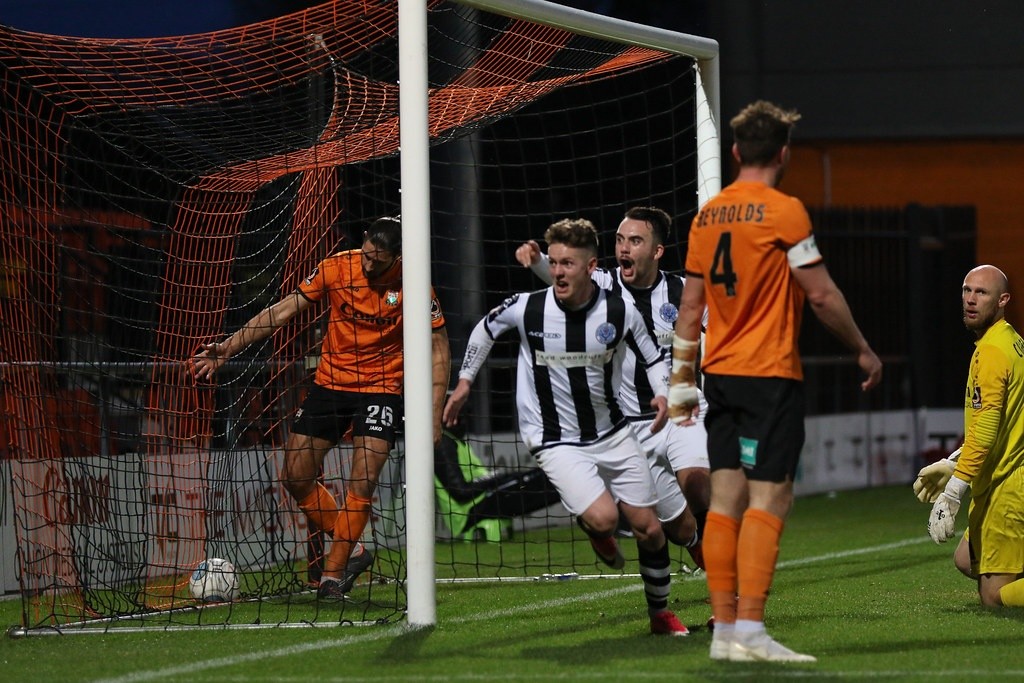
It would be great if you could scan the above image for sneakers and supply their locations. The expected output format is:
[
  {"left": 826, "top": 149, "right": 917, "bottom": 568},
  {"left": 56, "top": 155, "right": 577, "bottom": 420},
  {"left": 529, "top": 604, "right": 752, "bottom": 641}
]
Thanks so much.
[
  {"left": 317, "top": 580, "right": 345, "bottom": 603},
  {"left": 686, "top": 530, "right": 708, "bottom": 571},
  {"left": 710, "top": 637, "right": 734, "bottom": 660},
  {"left": 339, "top": 545, "right": 373, "bottom": 594},
  {"left": 650, "top": 611, "right": 692, "bottom": 637},
  {"left": 728, "top": 635, "right": 818, "bottom": 663},
  {"left": 577, "top": 516, "right": 626, "bottom": 570}
]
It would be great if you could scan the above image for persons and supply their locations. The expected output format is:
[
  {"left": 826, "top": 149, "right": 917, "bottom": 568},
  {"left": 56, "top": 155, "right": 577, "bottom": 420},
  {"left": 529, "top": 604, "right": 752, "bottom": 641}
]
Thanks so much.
[
  {"left": 443, "top": 218, "right": 690, "bottom": 637},
  {"left": 515, "top": 208, "right": 716, "bottom": 632},
  {"left": 666, "top": 102, "right": 883, "bottom": 664},
  {"left": 0, "top": 369, "right": 354, "bottom": 461},
  {"left": 914, "top": 264, "right": 1024, "bottom": 607},
  {"left": 184, "top": 218, "right": 451, "bottom": 605},
  {"left": 433, "top": 391, "right": 633, "bottom": 539}
]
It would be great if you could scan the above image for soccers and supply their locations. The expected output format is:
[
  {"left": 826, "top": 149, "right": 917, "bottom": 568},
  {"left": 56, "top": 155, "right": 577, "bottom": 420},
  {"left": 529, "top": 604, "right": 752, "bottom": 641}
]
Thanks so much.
[{"left": 189, "top": 557, "right": 239, "bottom": 607}]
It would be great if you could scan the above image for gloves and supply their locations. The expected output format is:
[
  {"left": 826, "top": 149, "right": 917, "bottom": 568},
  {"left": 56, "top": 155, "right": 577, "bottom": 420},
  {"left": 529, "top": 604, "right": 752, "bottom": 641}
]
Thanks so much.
[
  {"left": 913, "top": 459, "right": 963, "bottom": 503},
  {"left": 927, "top": 475, "right": 968, "bottom": 546}
]
